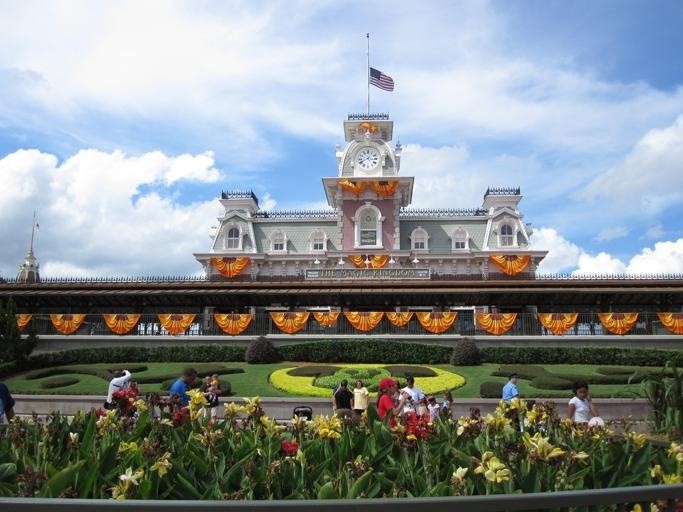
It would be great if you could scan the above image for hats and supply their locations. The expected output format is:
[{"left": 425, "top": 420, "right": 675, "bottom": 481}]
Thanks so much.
[
  {"left": 378, "top": 378, "right": 400, "bottom": 390},
  {"left": 588, "top": 416, "right": 604, "bottom": 428}
]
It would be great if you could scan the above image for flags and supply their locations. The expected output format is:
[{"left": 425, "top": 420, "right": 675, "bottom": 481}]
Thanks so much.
[{"left": 368, "top": 65, "right": 394, "bottom": 91}]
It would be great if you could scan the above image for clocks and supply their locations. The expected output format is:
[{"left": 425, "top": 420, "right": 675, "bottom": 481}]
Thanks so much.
[{"left": 356, "top": 147, "right": 381, "bottom": 170}]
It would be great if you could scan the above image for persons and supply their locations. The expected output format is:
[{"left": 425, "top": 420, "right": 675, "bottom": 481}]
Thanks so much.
[
  {"left": 170, "top": 368, "right": 198, "bottom": 408},
  {"left": 502, "top": 374, "right": 521, "bottom": 437},
  {"left": 566, "top": 379, "right": 599, "bottom": 426},
  {"left": 103, "top": 369, "right": 131, "bottom": 410},
  {"left": 0, "top": 381, "right": 17, "bottom": 426},
  {"left": 329, "top": 371, "right": 486, "bottom": 433},
  {"left": 208, "top": 373, "right": 219, "bottom": 403},
  {"left": 198, "top": 376, "right": 224, "bottom": 424}
]
